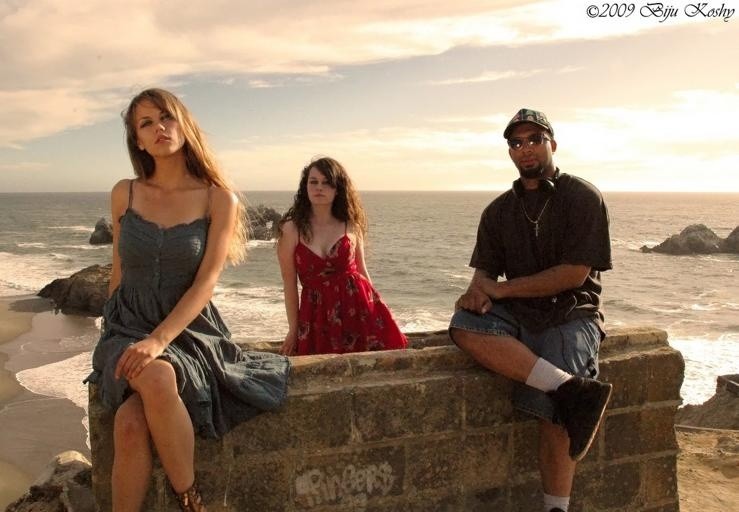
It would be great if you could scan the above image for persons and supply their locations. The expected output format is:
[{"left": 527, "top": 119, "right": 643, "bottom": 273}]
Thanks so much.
[
  {"left": 82, "top": 85, "right": 291, "bottom": 512},
  {"left": 449, "top": 107, "right": 616, "bottom": 511},
  {"left": 278, "top": 154, "right": 407, "bottom": 356}
]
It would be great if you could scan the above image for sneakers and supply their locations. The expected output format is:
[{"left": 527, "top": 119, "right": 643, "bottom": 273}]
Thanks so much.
[{"left": 544, "top": 374, "right": 613, "bottom": 462}]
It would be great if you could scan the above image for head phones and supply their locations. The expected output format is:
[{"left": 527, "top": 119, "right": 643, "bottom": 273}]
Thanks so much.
[{"left": 511, "top": 167, "right": 560, "bottom": 199}]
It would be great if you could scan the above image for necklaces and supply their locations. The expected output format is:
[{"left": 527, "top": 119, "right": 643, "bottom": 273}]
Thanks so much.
[{"left": 517, "top": 189, "right": 553, "bottom": 241}]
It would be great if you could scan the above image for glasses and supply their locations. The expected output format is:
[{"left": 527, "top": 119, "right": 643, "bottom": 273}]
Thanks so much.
[{"left": 507, "top": 133, "right": 550, "bottom": 152}]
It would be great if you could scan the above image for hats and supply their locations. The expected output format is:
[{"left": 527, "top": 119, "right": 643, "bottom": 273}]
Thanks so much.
[{"left": 504, "top": 109, "right": 554, "bottom": 139}]
